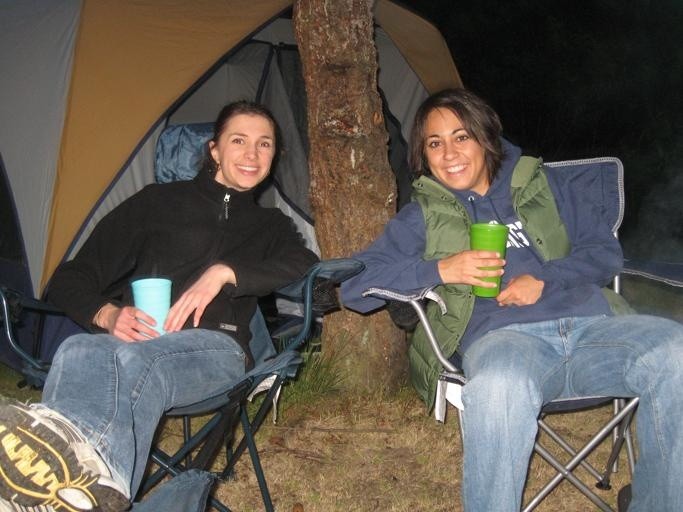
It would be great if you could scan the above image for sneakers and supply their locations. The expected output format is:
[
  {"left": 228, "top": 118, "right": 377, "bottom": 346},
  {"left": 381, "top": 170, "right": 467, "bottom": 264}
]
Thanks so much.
[{"left": 0, "top": 397, "right": 132, "bottom": 512}]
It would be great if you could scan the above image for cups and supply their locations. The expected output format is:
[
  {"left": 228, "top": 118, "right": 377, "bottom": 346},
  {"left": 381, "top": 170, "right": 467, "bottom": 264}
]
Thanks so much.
[
  {"left": 130, "top": 277, "right": 171, "bottom": 340},
  {"left": 471, "top": 223, "right": 508, "bottom": 297}
]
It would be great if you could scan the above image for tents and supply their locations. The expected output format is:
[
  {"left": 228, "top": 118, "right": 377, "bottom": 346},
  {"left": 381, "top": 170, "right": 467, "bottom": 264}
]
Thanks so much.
[{"left": 0, "top": 0, "right": 462, "bottom": 389}]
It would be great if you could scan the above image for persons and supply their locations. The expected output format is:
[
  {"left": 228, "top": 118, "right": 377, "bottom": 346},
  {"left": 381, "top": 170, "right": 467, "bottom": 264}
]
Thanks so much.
[
  {"left": 341, "top": 89, "right": 683, "bottom": 512},
  {"left": 0, "top": 101, "right": 321, "bottom": 512}
]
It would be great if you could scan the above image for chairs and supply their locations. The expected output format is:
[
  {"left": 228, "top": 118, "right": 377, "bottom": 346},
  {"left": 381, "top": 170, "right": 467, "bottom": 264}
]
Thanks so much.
[
  {"left": 2, "top": 123, "right": 365, "bottom": 512},
  {"left": 359, "top": 156, "right": 643, "bottom": 512}
]
